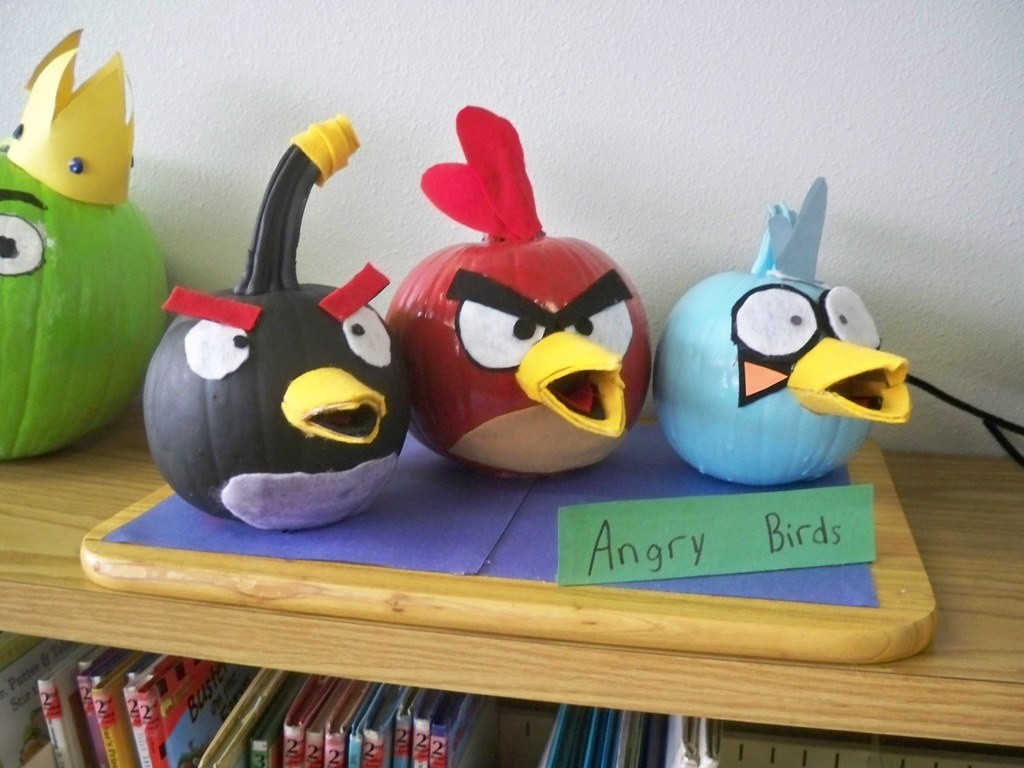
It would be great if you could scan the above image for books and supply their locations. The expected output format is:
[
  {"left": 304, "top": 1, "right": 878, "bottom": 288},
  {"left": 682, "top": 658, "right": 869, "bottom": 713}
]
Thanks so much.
[{"left": 1, "top": 638, "right": 724, "bottom": 768}]
